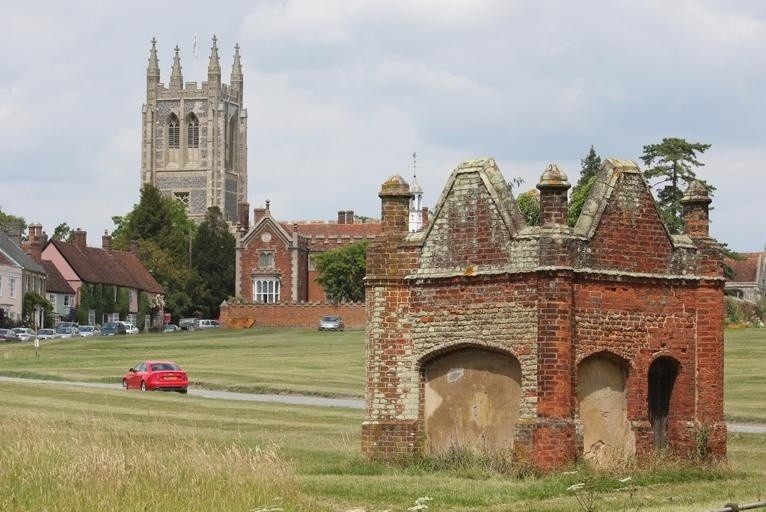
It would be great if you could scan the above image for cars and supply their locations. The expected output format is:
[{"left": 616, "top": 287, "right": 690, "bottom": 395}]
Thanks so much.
[
  {"left": 163, "top": 317, "right": 221, "bottom": 333},
  {"left": 0, "top": 318, "right": 139, "bottom": 343},
  {"left": 319, "top": 315, "right": 345, "bottom": 331},
  {"left": 122, "top": 360, "right": 189, "bottom": 393}
]
